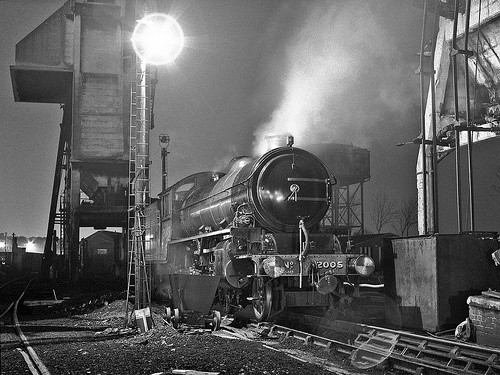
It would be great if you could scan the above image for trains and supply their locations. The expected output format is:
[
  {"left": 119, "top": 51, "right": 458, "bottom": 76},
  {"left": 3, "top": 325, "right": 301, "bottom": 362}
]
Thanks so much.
[{"left": 145, "top": 136, "right": 378, "bottom": 326}]
